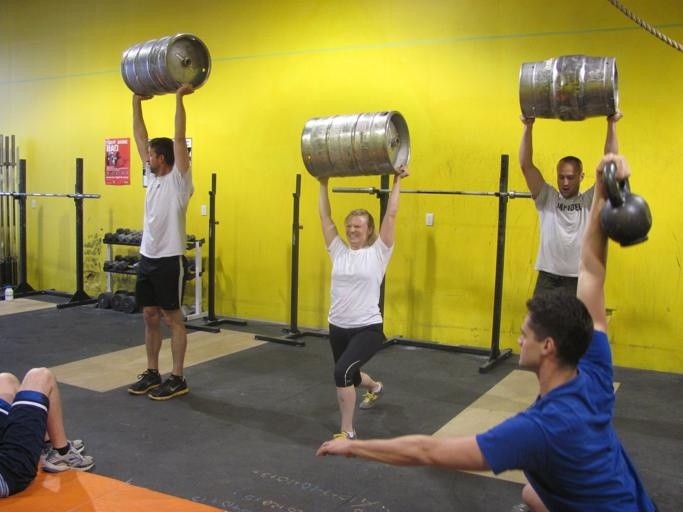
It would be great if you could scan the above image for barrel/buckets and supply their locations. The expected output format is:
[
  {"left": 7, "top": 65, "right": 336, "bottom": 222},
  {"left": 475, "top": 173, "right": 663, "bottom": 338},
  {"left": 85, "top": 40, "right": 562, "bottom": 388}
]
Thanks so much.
[
  {"left": 300, "top": 110, "right": 410, "bottom": 181},
  {"left": 121, "top": 33, "right": 210, "bottom": 96},
  {"left": 518, "top": 52, "right": 619, "bottom": 119}
]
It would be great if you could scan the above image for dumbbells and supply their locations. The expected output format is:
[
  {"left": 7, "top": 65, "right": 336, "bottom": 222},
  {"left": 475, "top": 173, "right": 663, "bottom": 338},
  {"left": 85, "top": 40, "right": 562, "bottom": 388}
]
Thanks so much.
[
  {"left": 98, "top": 290, "right": 138, "bottom": 314},
  {"left": 104, "top": 255, "right": 139, "bottom": 271},
  {"left": 186, "top": 264, "right": 204, "bottom": 280},
  {"left": 98, "top": 228, "right": 197, "bottom": 249}
]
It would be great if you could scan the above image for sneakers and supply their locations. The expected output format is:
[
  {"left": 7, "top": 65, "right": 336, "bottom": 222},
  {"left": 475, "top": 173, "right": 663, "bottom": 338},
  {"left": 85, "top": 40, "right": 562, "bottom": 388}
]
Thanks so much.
[
  {"left": 358, "top": 380, "right": 384, "bottom": 409},
  {"left": 40, "top": 437, "right": 96, "bottom": 473},
  {"left": 127, "top": 369, "right": 161, "bottom": 395},
  {"left": 148, "top": 375, "right": 189, "bottom": 400},
  {"left": 330, "top": 429, "right": 359, "bottom": 451}
]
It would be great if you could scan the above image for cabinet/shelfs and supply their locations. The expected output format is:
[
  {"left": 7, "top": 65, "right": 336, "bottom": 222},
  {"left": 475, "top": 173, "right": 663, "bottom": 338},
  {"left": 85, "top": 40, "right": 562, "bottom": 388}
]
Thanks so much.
[{"left": 102, "top": 228, "right": 208, "bottom": 321}]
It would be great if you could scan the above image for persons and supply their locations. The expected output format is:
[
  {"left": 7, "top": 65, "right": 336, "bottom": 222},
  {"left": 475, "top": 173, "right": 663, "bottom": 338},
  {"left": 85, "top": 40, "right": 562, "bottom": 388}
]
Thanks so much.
[
  {"left": 317, "top": 166, "right": 410, "bottom": 438},
  {"left": 128, "top": 85, "right": 194, "bottom": 401},
  {"left": 0, "top": 367, "right": 94, "bottom": 497},
  {"left": 519, "top": 112, "right": 623, "bottom": 300},
  {"left": 315, "top": 152, "right": 661, "bottom": 512}
]
